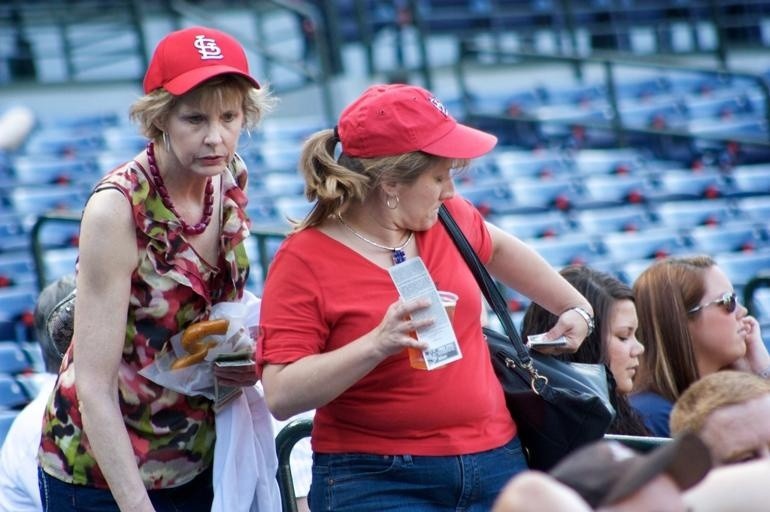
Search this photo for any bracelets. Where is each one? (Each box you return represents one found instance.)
[560,305,596,337]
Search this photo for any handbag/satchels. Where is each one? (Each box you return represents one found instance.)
[481,326,615,472]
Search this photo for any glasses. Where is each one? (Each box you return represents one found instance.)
[690,294,738,312]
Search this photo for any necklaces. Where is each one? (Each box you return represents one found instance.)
[337,208,413,266]
[146,141,215,236]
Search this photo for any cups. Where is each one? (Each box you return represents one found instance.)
[399,290,458,371]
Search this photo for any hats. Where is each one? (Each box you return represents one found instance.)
[143,27,261,97]
[33,275,76,361]
[553,429,714,510]
[336,84,498,160]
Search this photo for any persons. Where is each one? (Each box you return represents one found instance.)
[0,272,77,511]
[629,256,770,403]
[492,426,770,512]
[37,27,281,511]
[255,83,596,512]
[668,370,770,468]
[521,262,656,436]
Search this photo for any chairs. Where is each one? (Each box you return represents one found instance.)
[235,98,348,300]
